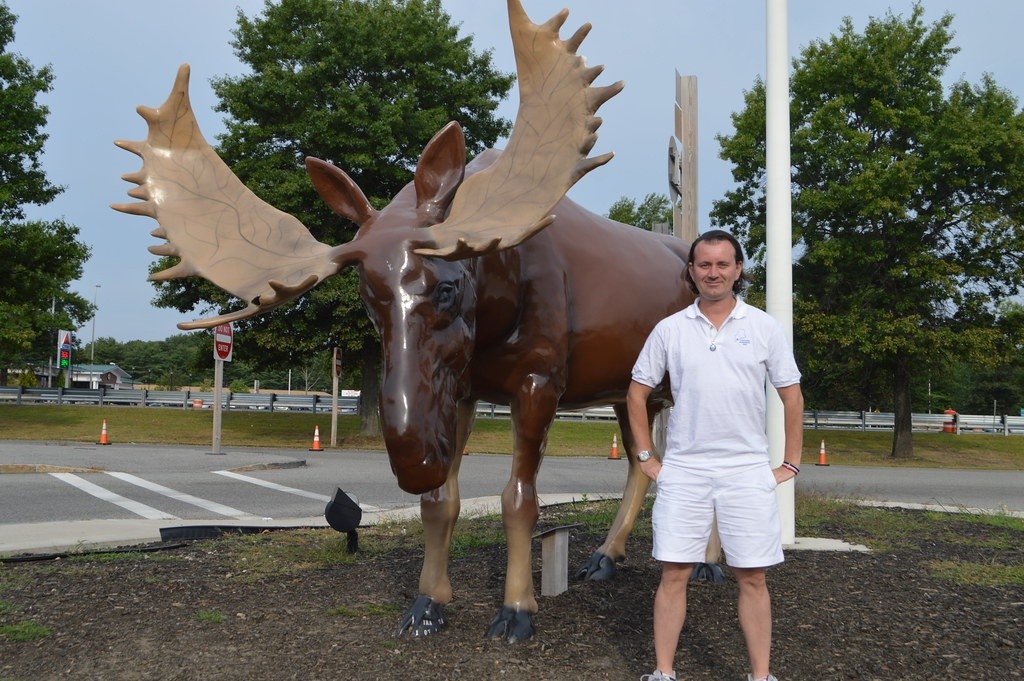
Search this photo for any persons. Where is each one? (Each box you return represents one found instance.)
[626,230,804,681]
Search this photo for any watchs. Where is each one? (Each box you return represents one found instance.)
[636,450,654,461]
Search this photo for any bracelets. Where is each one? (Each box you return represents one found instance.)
[781,461,799,475]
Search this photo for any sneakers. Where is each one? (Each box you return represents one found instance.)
[747,672,778,681]
[640,670,677,681]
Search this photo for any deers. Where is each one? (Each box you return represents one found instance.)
[108,1,742,646]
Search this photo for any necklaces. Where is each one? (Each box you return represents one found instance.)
[697,317,735,351]
